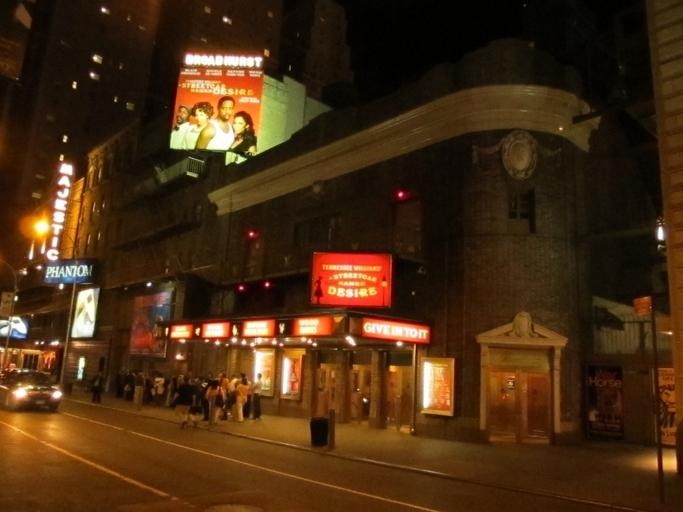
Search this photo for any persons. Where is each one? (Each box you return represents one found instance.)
[89,370,104,402]
[195,96,234,151]
[170,104,187,150]
[112,364,262,429]
[182,101,214,150]
[228,111,256,154]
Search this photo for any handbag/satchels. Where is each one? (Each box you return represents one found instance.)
[214,394,223,408]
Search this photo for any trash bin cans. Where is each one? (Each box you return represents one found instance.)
[310,417,328,446]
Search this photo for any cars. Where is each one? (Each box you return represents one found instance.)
[0,367,63,413]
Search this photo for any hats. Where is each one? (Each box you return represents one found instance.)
[178,105,191,113]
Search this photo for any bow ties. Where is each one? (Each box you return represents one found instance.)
[174,125,180,130]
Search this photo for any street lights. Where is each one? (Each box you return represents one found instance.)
[29,219,81,399]
[652,220,667,511]
